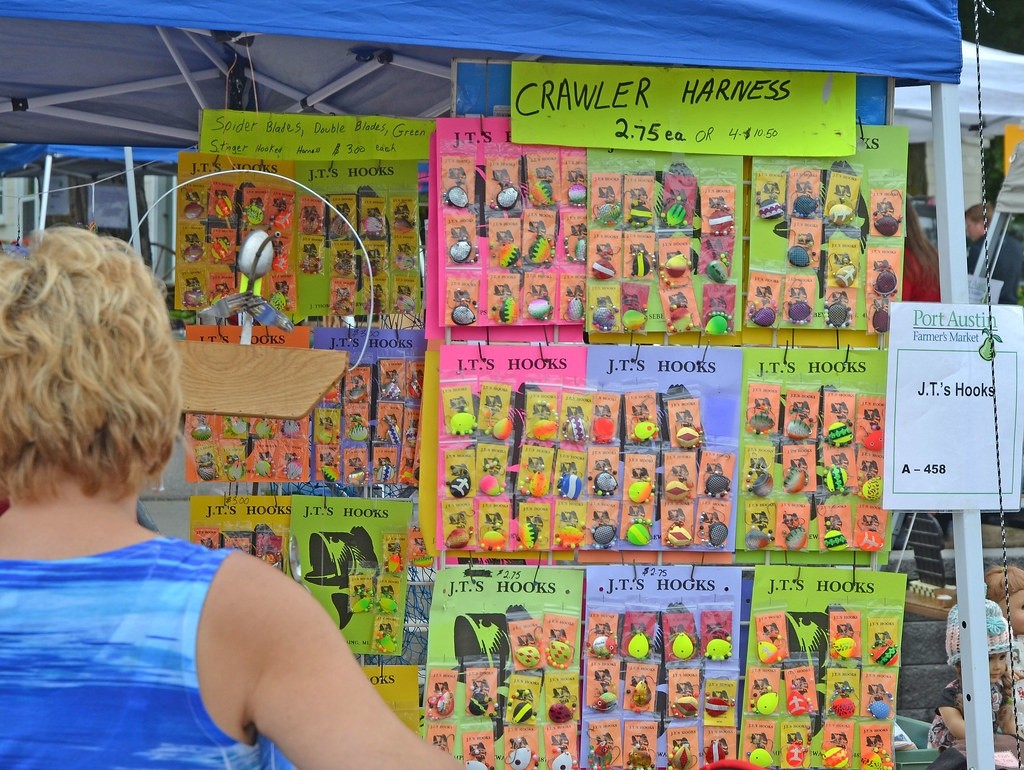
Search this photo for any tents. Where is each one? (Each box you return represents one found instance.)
[894,39,1024,143]
[0,0,995,770]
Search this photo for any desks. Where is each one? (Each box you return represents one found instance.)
[905,587,957,619]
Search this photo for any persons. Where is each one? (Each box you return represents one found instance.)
[927,583,1024,750]
[902,196,940,302]
[0,226,467,770]
[984,566,1024,735]
[965,202,1022,304]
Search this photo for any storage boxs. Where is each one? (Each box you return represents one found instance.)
[896,715,944,770]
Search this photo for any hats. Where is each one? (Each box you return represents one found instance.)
[944,583,1014,666]
